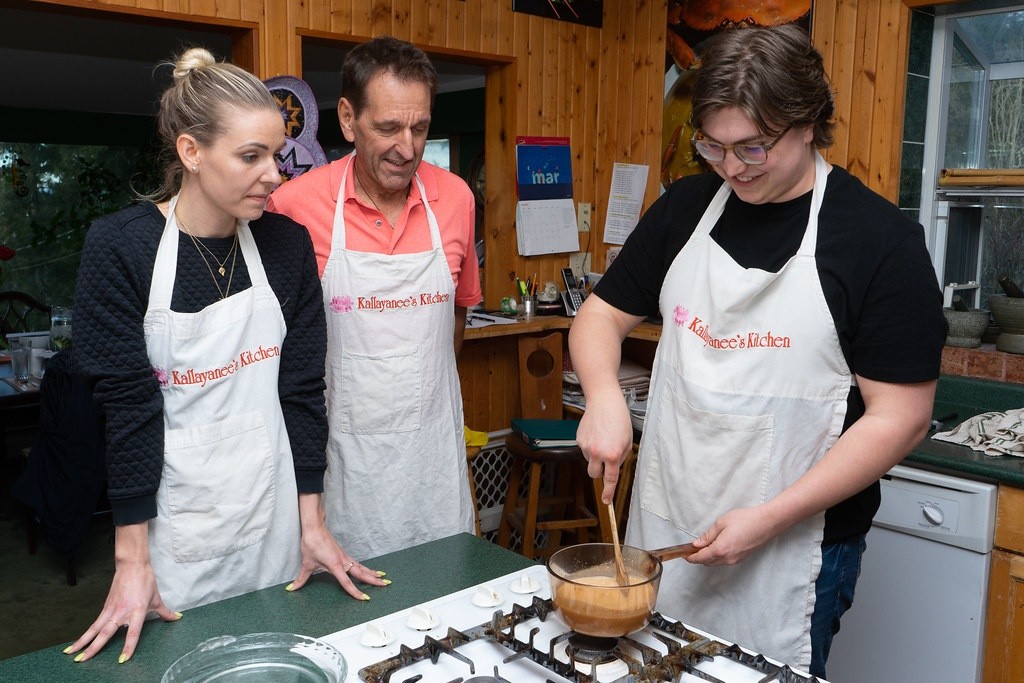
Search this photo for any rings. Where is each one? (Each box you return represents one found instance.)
[109,620,119,627]
[349,562,354,572]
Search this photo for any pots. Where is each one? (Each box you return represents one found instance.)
[544,543,704,637]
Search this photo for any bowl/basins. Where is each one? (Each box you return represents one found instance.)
[159,630,347,683]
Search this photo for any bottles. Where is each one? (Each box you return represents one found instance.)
[48,305,74,351]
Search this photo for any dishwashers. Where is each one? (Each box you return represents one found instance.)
[823,465,997,683]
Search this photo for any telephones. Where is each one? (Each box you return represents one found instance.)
[559,268,589,317]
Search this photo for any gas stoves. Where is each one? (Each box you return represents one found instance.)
[288,562,832,683]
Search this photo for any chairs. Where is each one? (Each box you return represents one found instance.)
[7,345,119,587]
[0,290,54,352]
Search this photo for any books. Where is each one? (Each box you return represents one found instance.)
[562,358,650,405]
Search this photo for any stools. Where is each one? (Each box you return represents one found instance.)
[465,444,483,537]
[495,432,615,563]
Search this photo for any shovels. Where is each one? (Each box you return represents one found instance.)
[601,472,629,597]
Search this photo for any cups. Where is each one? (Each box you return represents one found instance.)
[522,301,536,317]
[9,338,33,383]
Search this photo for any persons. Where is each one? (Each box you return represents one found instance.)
[568,26,950,678]
[61,41,392,662]
[264,37,484,562]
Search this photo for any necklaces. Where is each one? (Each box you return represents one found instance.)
[176,209,238,300]
[353,160,381,213]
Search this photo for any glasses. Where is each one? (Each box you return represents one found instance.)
[464,314,495,326]
[689,124,794,166]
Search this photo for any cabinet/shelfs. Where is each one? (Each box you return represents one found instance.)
[978,485,1024,683]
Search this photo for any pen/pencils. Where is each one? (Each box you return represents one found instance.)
[533,283,538,296]
[526,276,531,289]
[530,273,537,296]
[516,278,523,295]
[470,315,496,322]
[575,274,595,291]
